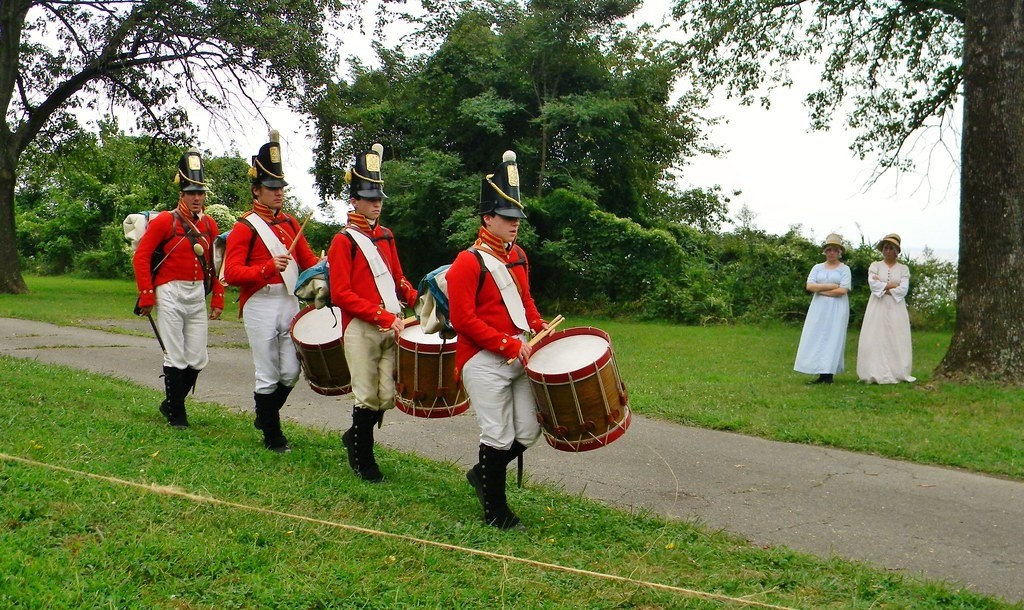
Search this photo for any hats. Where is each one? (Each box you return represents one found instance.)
[477,150,527,220]
[247,129,289,189]
[822,233,845,253]
[877,233,901,254]
[345,142,389,200]
[174,147,210,192]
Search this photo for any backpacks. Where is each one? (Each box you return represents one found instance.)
[293,225,390,310]
[119,210,210,261]
[212,214,295,288]
[414,245,526,341]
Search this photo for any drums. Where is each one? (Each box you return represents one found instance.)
[289,303,352,396]
[392,315,472,418]
[521,326,632,452]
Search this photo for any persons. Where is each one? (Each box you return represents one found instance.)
[446,150,555,529]
[793,232,851,385]
[224,131,319,453]
[856,233,916,385]
[132,147,224,428]
[327,144,420,484]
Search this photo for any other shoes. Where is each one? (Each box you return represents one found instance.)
[807,373,834,386]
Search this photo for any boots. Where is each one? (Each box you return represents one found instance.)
[158,366,202,419]
[465,440,527,508]
[342,409,385,473]
[162,365,191,428]
[479,444,520,531]
[352,404,386,484]
[254,392,290,452]
[254,381,294,430]
